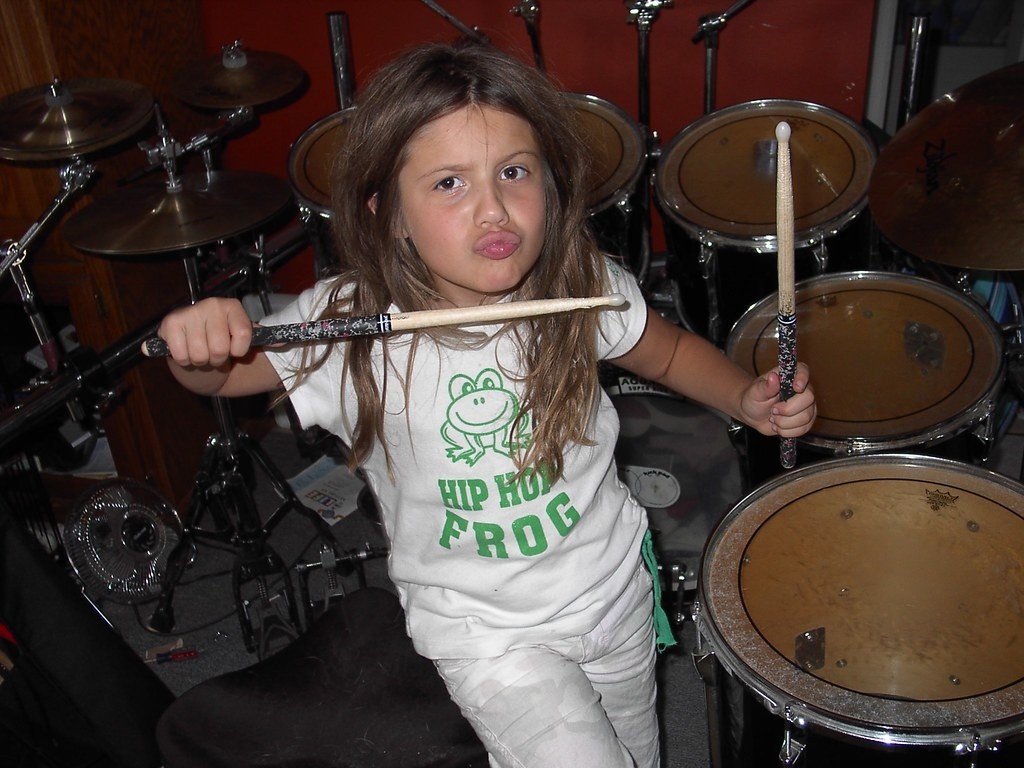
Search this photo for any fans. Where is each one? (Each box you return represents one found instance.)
[64,479,189,606]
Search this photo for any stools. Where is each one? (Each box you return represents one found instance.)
[155,588,491,768]
[610,392,741,768]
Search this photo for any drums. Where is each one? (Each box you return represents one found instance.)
[722,270,1009,496]
[689,453,1024,768]
[648,97,881,353]
[286,103,368,277]
[553,89,664,286]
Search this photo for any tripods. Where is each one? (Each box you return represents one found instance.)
[146,247,354,635]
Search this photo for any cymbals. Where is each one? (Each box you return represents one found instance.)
[866,58,1024,271]
[61,166,293,256]
[0,79,158,162]
[170,47,307,110]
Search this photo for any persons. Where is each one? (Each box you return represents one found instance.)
[157,43,819,768]
